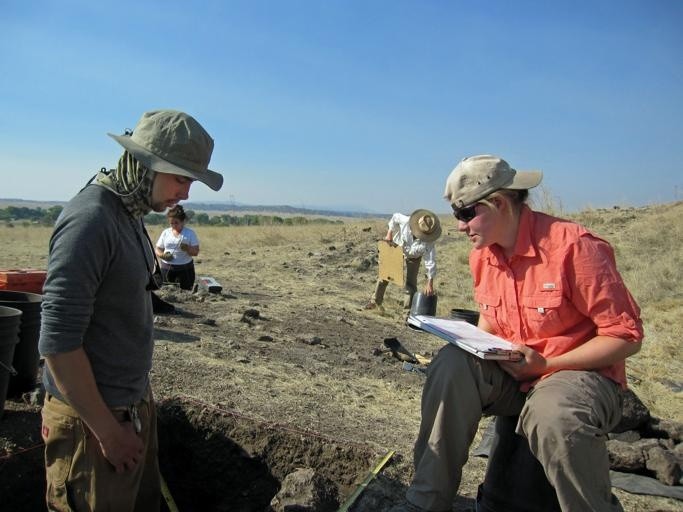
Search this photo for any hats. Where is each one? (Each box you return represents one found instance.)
[408,209,442,242]
[444,155,543,210]
[107,109,224,192]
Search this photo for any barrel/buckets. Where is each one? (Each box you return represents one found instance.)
[412,290,437,319]
[475,415,559,511]
[0,305,22,430]
[1,289,45,397]
[450,309,479,327]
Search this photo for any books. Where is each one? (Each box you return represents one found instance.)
[406,313,524,361]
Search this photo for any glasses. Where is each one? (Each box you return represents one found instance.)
[454,197,489,222]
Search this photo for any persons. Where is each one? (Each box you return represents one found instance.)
[153,204,200,291]
[391,154,644,512]
[363,209,442,309]
[36,109,223,512]
